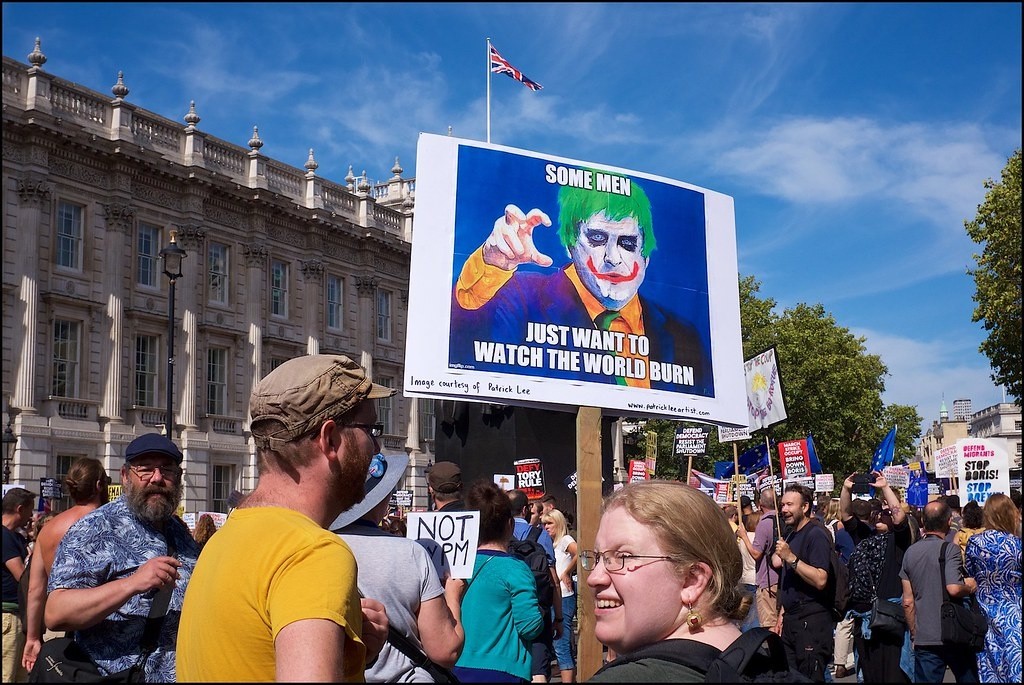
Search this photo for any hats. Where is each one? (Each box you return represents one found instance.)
[428,461,462,493]
[327,451,409,531]
[125,432,183,465]
[249,354,400,452]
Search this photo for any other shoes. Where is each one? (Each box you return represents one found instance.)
[833,664,855,678]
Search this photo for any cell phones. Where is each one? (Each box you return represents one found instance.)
[853,474,876,485]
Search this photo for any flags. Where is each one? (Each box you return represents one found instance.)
[714,438,770,480]
[868,427,895,496]
[806,433,823,473]
[488,42,544,92]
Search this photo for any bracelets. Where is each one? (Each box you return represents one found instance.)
[791,557,799,569]
[555,618,564,623]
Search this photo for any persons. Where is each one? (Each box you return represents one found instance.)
[378,505,407,538]
[2,456,111,684]
[583,480,814,684]
[329,452,466,684]
[43,432,200,683]
[428,461,577,683]
[192,514,217,551]
[723,470,1022,684]
[175,355,401,684]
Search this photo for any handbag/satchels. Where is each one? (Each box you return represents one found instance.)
[940,600,988,654]
[27,637,146,685]
[867,595,907,641]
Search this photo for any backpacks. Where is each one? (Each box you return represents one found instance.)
[785,520,855,622]
[761,513,792,598]
[505,526,554,608]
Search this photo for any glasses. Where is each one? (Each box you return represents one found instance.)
[93,477,112,485]
[518,503,534,514]
[310,421,386,440]
[579,550,689,571]
[127,466,183,481]
[873,508,892,515]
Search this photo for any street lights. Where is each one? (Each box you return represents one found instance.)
[2,420,18,484]
[159,229,189,441]
